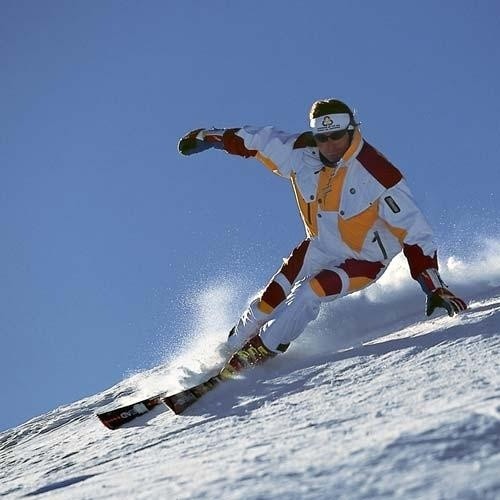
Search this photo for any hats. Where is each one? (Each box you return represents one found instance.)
[310,99,355,136]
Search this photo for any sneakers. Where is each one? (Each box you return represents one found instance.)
[219,335,278,381]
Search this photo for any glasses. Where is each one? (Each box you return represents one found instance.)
[315,129,347,143]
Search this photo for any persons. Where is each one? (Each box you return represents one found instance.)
[179,100,469,394]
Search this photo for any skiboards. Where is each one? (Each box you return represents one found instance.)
[97,373,227,430]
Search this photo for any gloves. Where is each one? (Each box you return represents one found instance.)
[417,267,467,317]
[178,128,213,155]
